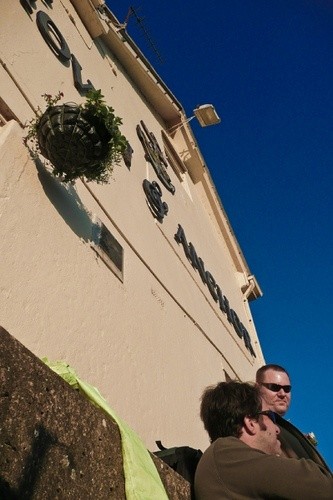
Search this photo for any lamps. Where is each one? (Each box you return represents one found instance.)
[167,103,220,135]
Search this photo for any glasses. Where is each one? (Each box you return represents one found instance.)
[260,382,292,393]
[256,409,276,424]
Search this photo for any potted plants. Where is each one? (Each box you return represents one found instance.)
[21,86,132,185]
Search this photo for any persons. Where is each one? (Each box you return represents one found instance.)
[192,382,333,500]
[253,364,332,480]
